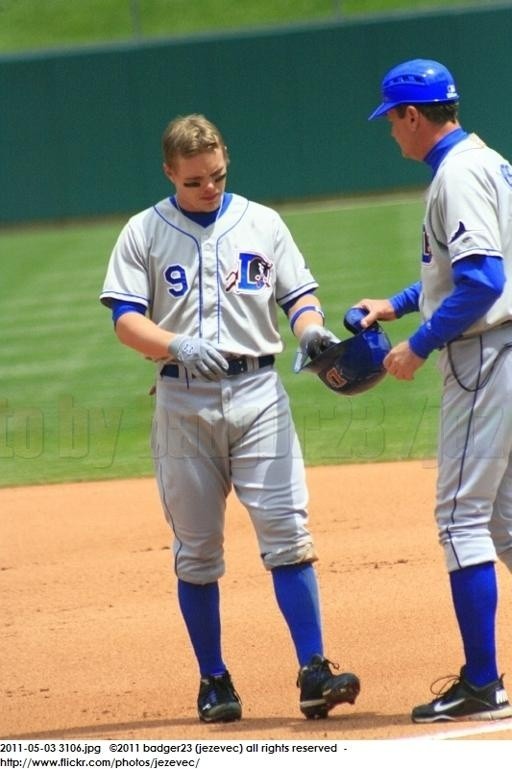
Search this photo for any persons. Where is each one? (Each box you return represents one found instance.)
[351,55,511,723]
[101,113,362,723]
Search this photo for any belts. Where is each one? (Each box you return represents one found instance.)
[157,355,274,382]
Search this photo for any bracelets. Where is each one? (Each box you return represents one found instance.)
[289,304,326,336]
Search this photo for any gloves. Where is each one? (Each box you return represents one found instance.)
[291,321,342,375]
[166,332,229,385]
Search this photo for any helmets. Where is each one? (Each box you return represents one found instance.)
[294,308,392,397]
[367,55,459,122]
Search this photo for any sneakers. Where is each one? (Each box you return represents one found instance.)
[195,671,243,722]
[410,663,512,722]
[293,653,361,720]
